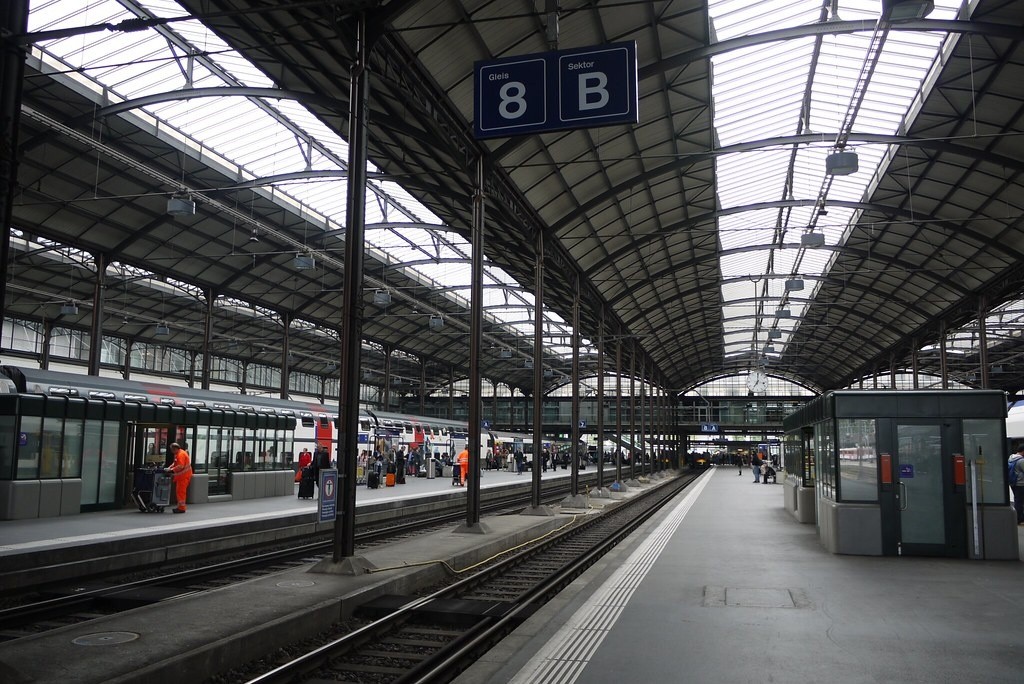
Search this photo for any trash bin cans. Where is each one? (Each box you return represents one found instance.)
[131,461,174,513]
[452,458,461,486]
[426,457,436,479]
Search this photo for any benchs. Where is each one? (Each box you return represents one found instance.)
[442,466,453,477]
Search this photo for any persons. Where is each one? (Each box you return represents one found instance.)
[440,452,450,467]
[751,450,776,484]
[405,446,425,477]
[486,447,509,471]
[373,445,384,488]
[457,447,468,486]
[604,451,657,465]
[387,445,397,474]
[396,446,407,484]
[307,443,331,489]
[298,448,312,469]
[542,448,570,472]
[712,452,750,466]
[147,443,154,455]
[164,443,193,513]
[514,447,523,475]
[737,453,743,475]
[1008,445,1024,525]
[360,450,372,463]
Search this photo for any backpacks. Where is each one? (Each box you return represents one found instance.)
[1008,457,1024,485]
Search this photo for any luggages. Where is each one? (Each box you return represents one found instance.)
[298,466,314,499]
[386,463,395,486]
[367,462,378,489]
[561,461,566,468]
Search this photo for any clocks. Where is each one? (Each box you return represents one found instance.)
[746,370,769,393]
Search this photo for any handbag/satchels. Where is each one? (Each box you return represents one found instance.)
[758,459,763,466]
[295,470,302,482]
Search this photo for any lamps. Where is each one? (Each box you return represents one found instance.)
[441,389,448,394]
[373,290,391,305]
[154,323,170,336]
[757,228,826,366]
[294,250,315,270]
[360,369,368,379]
[586,386,592,393]
[560,376,569,383]
[544,369,553,376]
[429,315,444,328]
[880,0,935,24]
[826,149,859,175]
[524,359,534,367]
[288,353,293,361]
[227,342,239,350]
[327,363,337,371]
[501,348,511,358]
[393,377,401,385]
[166,192,195,216]
[61,302,79,316]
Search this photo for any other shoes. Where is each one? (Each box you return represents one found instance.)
[761,482,768,484]
[460,484,464,487]
[1017,520,1024,526]
[753,481,759,483]
[172,508,185,513]
